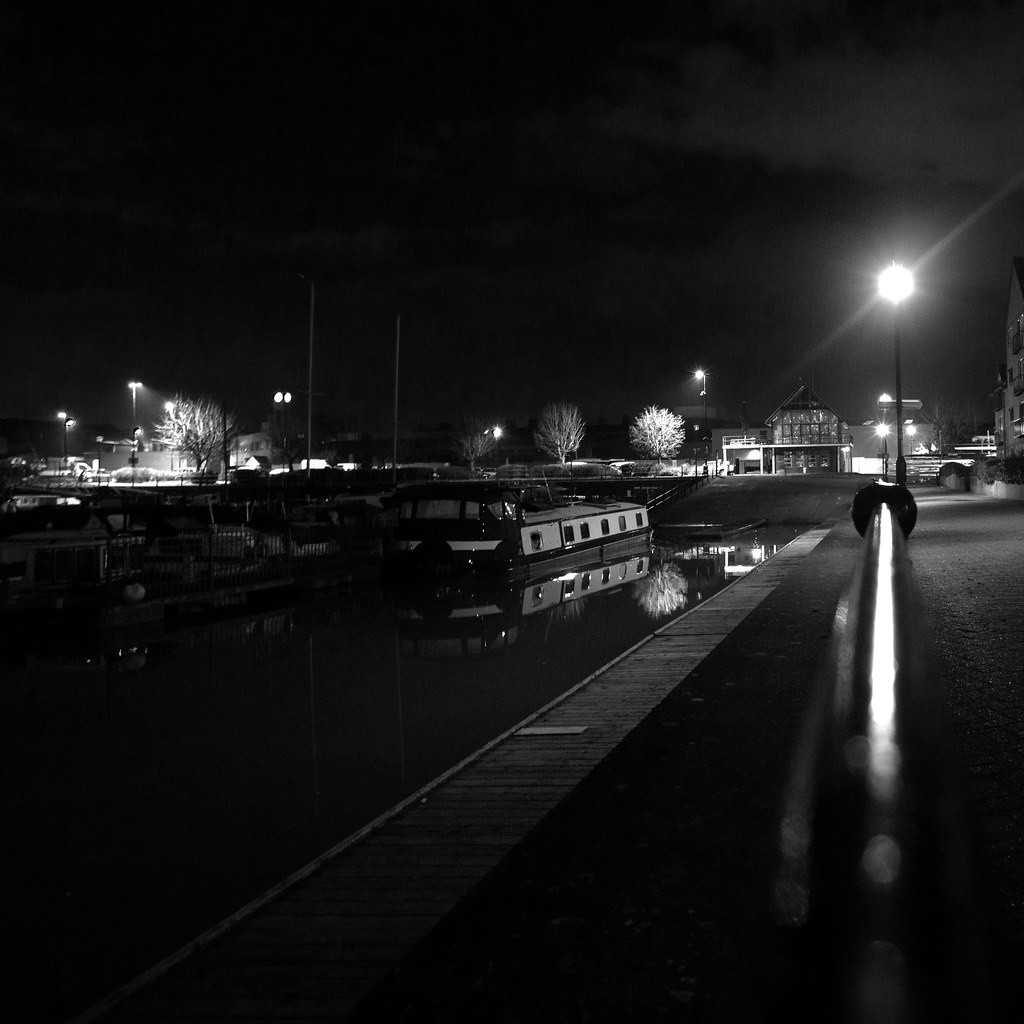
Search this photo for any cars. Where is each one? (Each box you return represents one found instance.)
[480,467,498,480]
[81,467,112,483]
[698,460,722,476]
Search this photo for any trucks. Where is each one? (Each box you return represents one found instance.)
[38,462,91,479]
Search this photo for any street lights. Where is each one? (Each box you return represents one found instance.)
[491,428,503,479]
[694,371,709,475]
[273,391,293,464]
[876,423,889,482]
[64,418,76,456]
[128,382,143,484]
[906,426,916,458]
[96,435,104,484]
[867,244,922,487]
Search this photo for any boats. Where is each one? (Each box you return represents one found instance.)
[0,473,655,608]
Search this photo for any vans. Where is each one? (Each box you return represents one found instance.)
[301,459,332,470]
[607,462,635,469]
